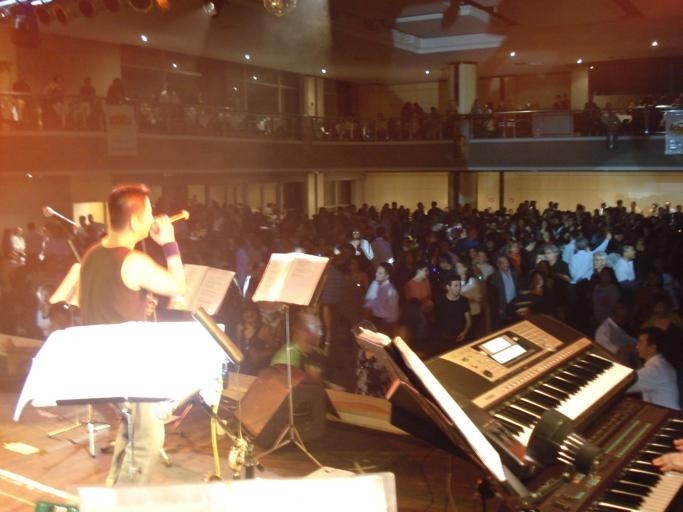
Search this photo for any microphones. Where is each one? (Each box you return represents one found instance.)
[42,205,81,231]
[151,208,189,231]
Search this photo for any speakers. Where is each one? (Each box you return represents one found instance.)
[233,362,328,444]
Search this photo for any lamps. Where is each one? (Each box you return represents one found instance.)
[263,0,298,17]
[0,0,228,34]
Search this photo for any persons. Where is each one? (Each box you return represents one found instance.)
[623,327,679,412]
[653,437,682,475]
[2,186,682,386]
[325,93,681,136]
[73,184,192,488]
[1,65,302,137]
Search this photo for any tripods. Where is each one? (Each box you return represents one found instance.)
[47,404,116,458]
[253,310,324,472]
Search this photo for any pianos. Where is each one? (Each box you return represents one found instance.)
[420,317,683,512]
